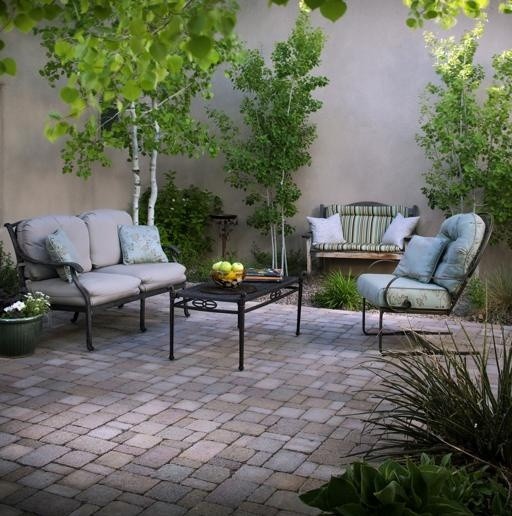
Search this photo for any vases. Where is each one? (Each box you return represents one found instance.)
[0,313,44,357]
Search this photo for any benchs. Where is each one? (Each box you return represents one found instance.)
[2,207,191,353]
[301,200,422,280]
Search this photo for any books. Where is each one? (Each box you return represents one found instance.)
[242,268,284,283]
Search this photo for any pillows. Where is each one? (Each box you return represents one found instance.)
[379,211,422,251]
[305,212,348,246]
[116,223,170,267]
[391,234,450,285]
[46,223,83,283]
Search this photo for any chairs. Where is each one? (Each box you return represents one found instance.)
[356,211,495,357]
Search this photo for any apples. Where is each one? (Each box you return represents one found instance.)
[211,261,244,280]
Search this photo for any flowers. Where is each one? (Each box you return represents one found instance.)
[0,241,50,317]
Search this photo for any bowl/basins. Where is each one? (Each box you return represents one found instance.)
[210,270,247,289]
